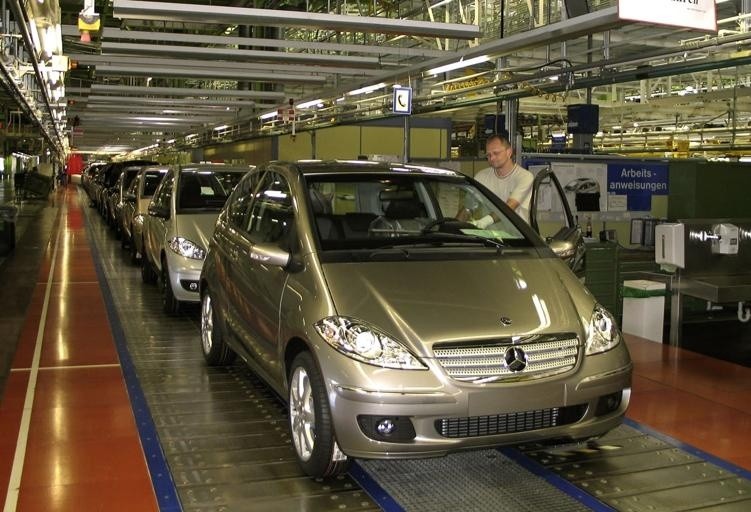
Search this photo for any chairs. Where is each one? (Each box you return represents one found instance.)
[368,189,440,239]
[285,190,341,252]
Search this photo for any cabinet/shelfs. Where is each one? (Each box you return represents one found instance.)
[574,245,706,324]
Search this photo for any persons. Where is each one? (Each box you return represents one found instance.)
[455,133,533,239]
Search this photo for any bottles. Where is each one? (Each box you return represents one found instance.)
[586,216,593,238]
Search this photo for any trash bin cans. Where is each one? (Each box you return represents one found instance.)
[0,206,17,256]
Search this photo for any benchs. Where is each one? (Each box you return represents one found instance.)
[265,212,386,240]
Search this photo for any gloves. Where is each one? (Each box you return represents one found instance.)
[466,214,495,231]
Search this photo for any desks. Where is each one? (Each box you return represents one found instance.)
[634,268,751,347]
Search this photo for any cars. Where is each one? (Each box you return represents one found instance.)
[117,165,172,263]
[81,160,160,240]
[200,156,634,482]
[147,164,333,314]
